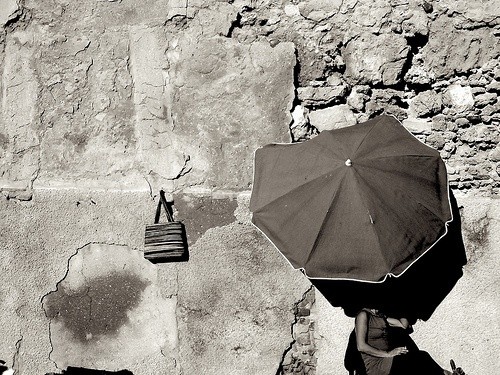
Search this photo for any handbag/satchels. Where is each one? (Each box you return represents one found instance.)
[140,189,189,262]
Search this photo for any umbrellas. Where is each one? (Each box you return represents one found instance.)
[246,111,453,284]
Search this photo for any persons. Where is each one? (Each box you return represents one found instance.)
[355,285,466,375]
